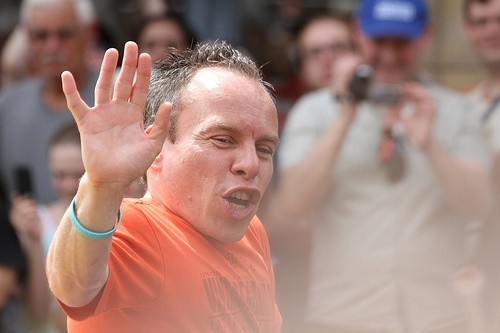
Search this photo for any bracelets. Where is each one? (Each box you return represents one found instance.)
[69,199,121,238]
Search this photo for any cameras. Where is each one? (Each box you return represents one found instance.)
[336,60,404,104]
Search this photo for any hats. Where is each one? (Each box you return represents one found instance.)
[359,0,429,38]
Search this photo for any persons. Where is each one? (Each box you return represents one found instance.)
[0,0,500,333]
